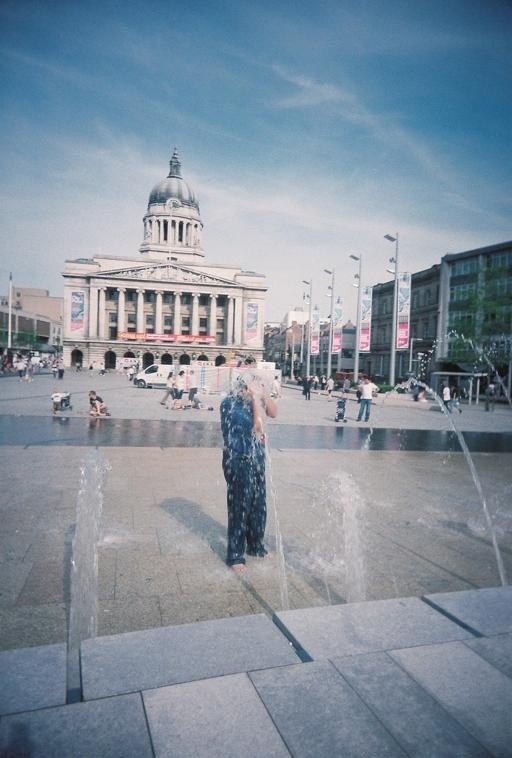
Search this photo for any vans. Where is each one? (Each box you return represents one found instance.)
[132,363,190,390]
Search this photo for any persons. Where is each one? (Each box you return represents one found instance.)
[137,360,141,372]
[160,372,176,406]
[439,381,464,413]
[88,390,112,416]
[295,373,334,402]
[412,385,425,402]
[88,361,97,377]
[75,359,82,376]
[51,390,72,415]
[218,357,281,577]
[334,375,380,423]
[122,359,139,381]
[185,370,198,409]
[1,353,65,384]
[166,369,185,410]
[99,362,105,376]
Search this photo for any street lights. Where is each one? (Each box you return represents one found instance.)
[283,328,293,374]
[382,229,401,388]
[348,251,363,380]
[322,265,337,380]
[301,278,313,377]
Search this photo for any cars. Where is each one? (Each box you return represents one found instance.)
[335,370,369,380]
